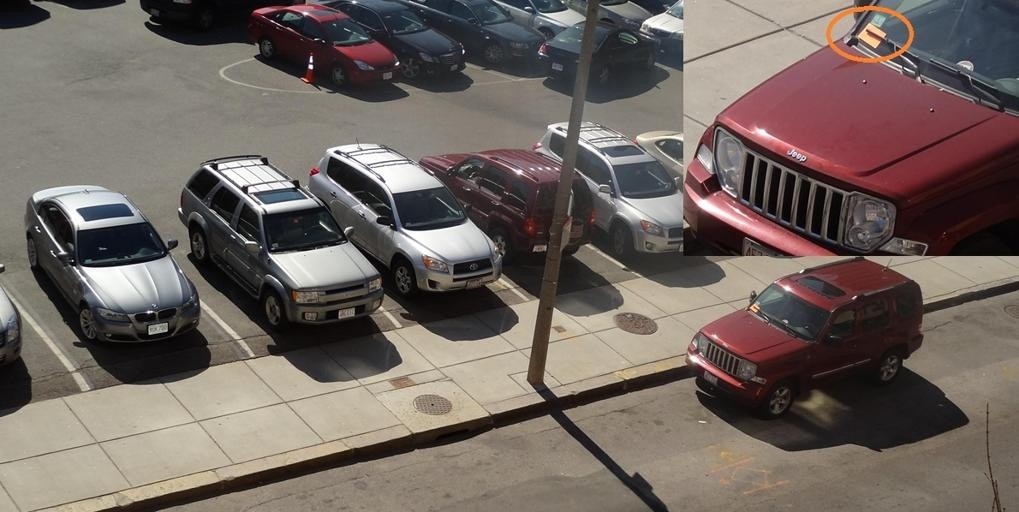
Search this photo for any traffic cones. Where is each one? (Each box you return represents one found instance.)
[301,52,319,83]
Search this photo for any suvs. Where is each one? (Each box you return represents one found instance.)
[684,2,1017,258]
[688,257,925,421]
[177,153,385,330]
[531,117,685,266]
[310,136,504,299]
[418,147,594,264]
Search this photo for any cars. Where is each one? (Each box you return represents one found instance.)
[635,128,683,184]
[138,0,240,39]
[538,20,662,87]
[562,0,653,31]
[246,2,402,88]
[639,1,683,59]
[22,183,201,347]
[494,0,587,39]
[406,0,547,70]
[0,262,23,368]
[323,0,468,83]
